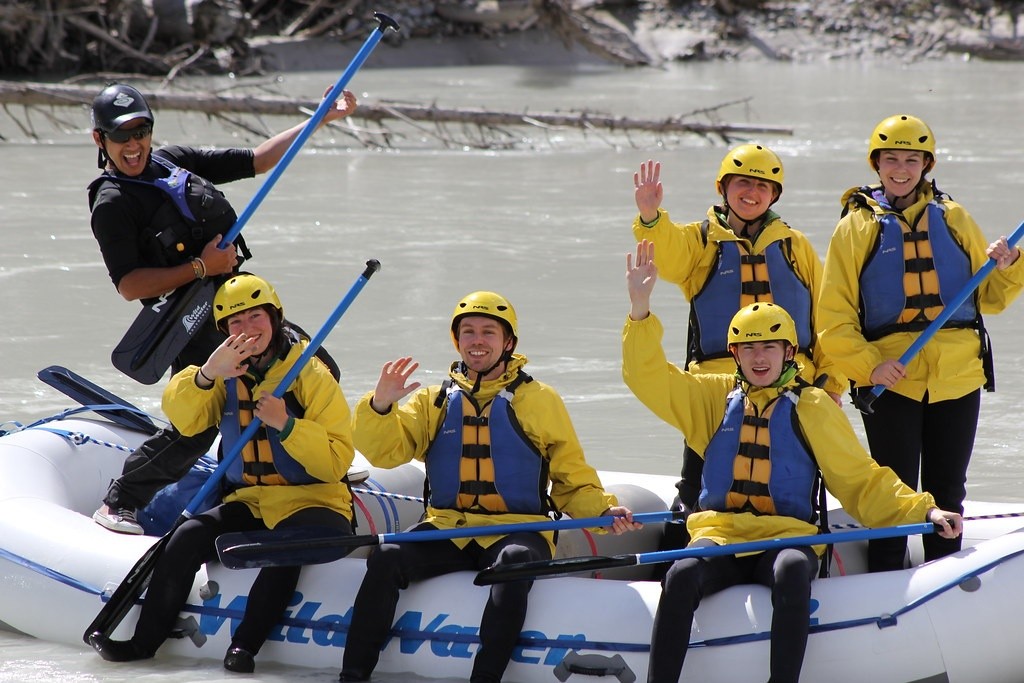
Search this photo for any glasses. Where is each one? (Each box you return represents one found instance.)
[100,123,151,143]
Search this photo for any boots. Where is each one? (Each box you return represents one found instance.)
[89,603,168,662]
[224,612,274,672]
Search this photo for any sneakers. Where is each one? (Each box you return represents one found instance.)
[93,503,144,535]
[346,464,369,483]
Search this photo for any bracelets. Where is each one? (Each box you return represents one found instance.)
[190,260,201,280]
[199,368,215,381]
[194,257,206,280]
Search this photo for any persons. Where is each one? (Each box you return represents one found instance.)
[89,276,357,673]
[817,113,1023,573]
[618,238,963,683]
[633,141,848,584]
[86,80,357,534]
[339,290,644,682]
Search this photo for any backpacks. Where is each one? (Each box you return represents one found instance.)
[86,152,253,261]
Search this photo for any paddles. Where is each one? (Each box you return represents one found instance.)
[110,11,402,387]
[215,511,686,570]
[36,365,218,465]
[474,519,957,586]
[82,259,381,646]
[853,220,1024,416]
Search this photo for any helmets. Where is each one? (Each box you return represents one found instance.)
[867,114,937,173]
[449,291,519,356]
[91,84,154,133]
[715,144,784,197]
[213,274,284,334]
[726,302,799,357]
